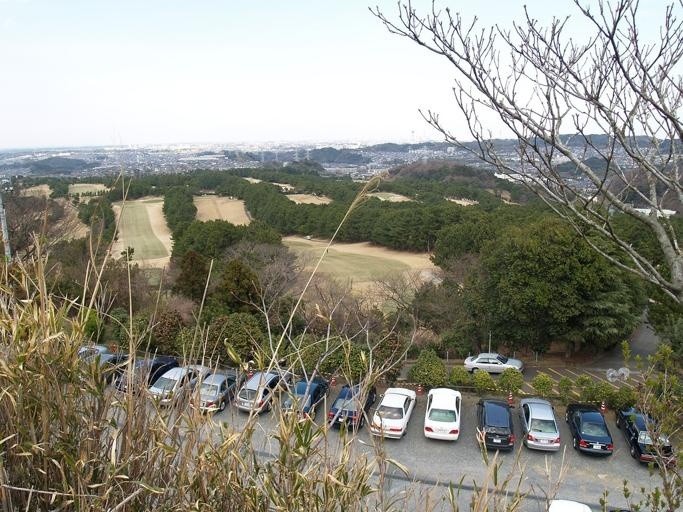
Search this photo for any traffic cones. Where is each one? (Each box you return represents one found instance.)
[508,390,515,408]
[601,399,606,413]
[416,382,425,395]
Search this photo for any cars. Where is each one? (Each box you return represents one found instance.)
[564,401,614,458]
[518,397,561,453]
[463,352,524,375]
[81,345,248,416]
[279,376,330,423]
[475,398,515,451]
[328,383,378,433]
[615,405,677,468]
[422,387,462,440]
[369,387,417,440]
[233,366,292,416]
